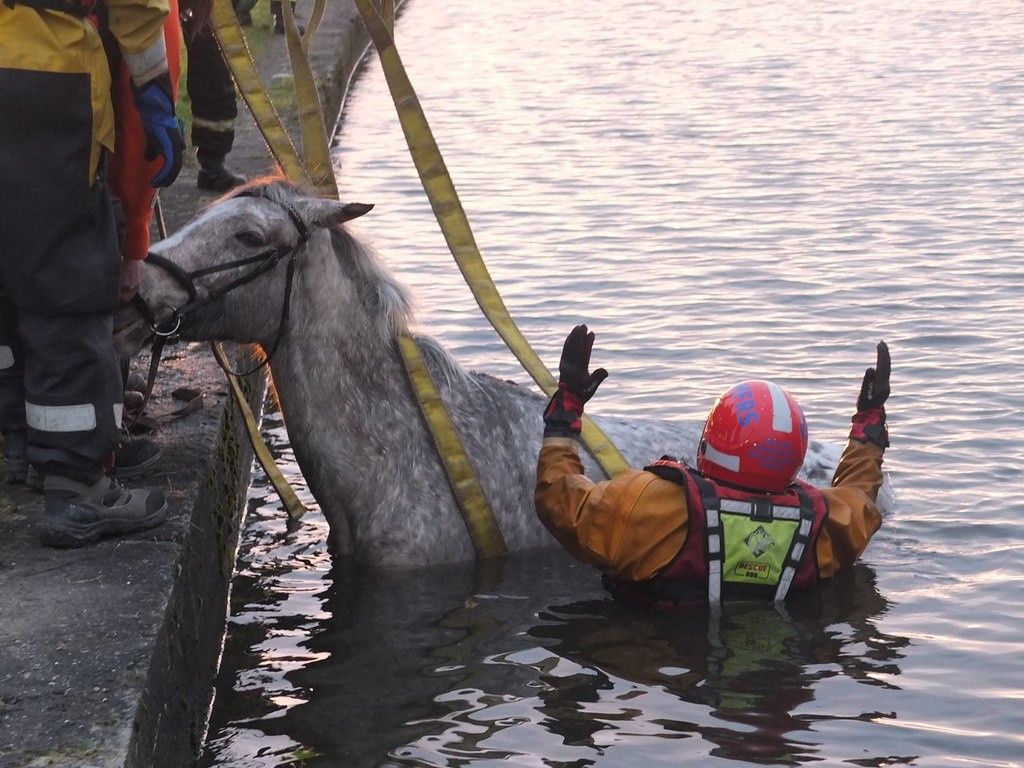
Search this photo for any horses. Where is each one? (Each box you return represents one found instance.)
[112,176,897,566]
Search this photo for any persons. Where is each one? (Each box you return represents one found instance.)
[0,0,304,547]
[533,325,891,620]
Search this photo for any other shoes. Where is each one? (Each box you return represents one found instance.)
[123,390,145,408]
[274,16,304,35]
[197,163,246,191]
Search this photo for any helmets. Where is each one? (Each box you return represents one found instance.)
[697,380,809,494]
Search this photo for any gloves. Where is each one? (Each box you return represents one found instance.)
[543,324,609,432]
[847,341,891,449]
[130,68,187,189]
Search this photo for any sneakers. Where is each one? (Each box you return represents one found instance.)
[39,475,168,547]
[1,455,25,483]
[26,438,165,492]
